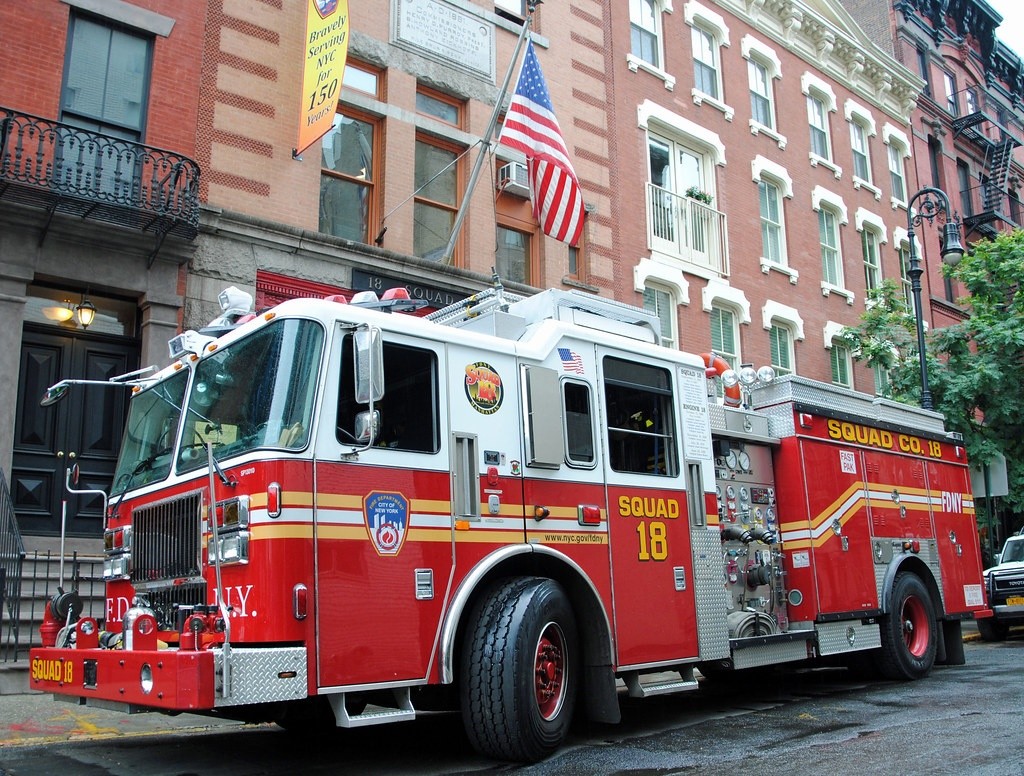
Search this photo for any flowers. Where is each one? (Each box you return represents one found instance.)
[685,185,714,204]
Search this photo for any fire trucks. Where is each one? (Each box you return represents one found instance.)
[30,265,995,766]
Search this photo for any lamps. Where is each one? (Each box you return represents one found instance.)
[76,286,97,331]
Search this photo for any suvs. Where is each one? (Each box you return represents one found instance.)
[974,534,1023,641]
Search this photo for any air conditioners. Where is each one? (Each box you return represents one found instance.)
[497,162,531,198]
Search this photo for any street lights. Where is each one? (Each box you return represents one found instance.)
[907,184,965,412]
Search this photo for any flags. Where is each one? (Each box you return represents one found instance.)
[493,38,584,250]
